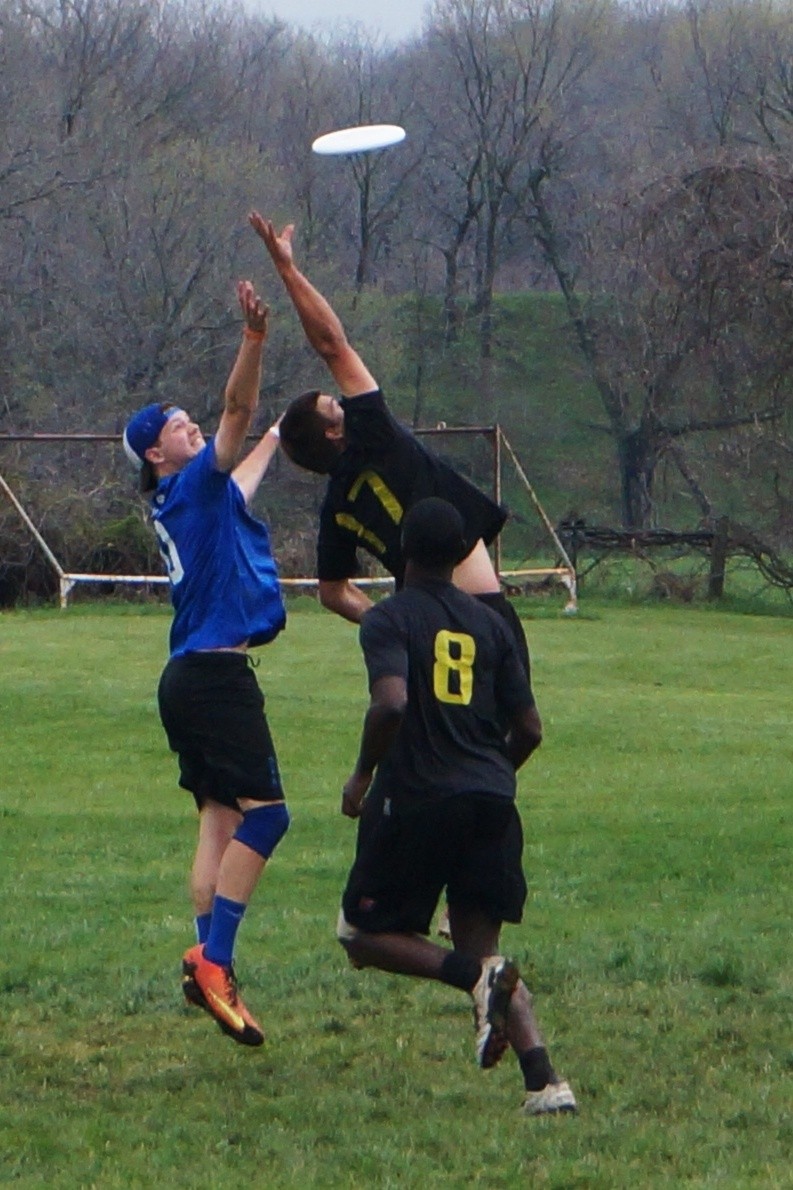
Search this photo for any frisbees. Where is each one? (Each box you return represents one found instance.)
[311,123,407,155]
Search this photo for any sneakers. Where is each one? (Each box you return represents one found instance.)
[472,951,522,1069]
[519,1079,580,1121]
[180,947,267,1048]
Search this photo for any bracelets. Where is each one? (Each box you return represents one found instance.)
[242,326,267,340]
[269,426,281,438]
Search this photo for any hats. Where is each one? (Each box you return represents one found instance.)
[401,496,466,590]
[121,398,185,493]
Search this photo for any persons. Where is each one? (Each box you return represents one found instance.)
[336,490,578,1113]
[247,209,532,943]
[124,272,288,1045]
